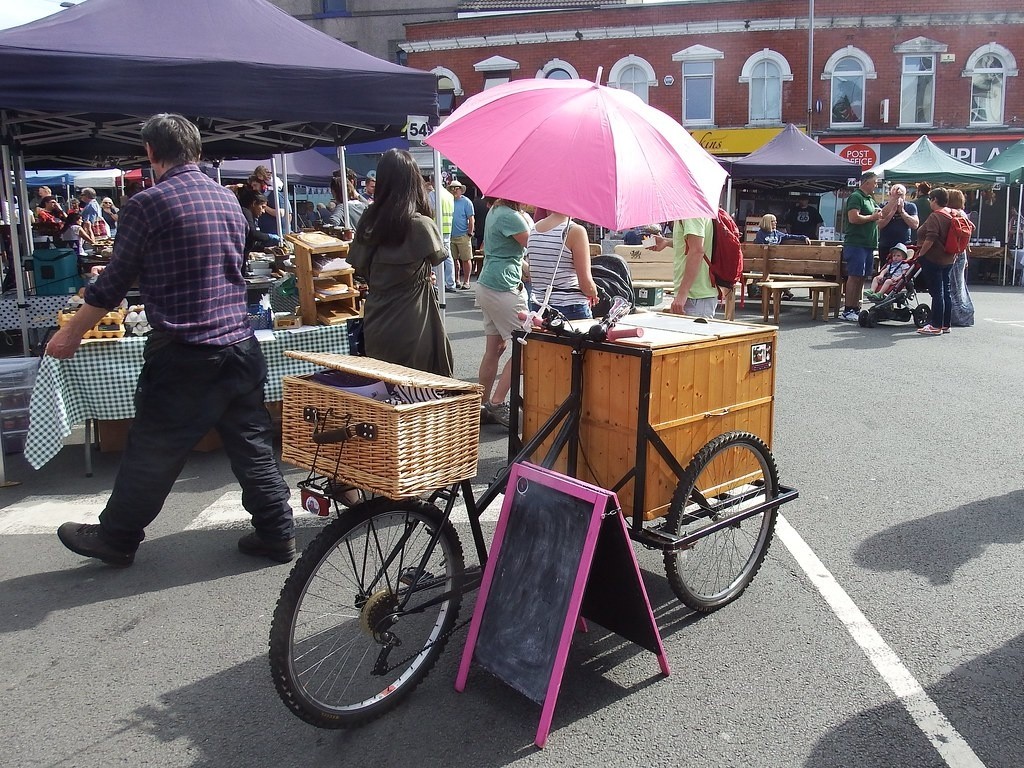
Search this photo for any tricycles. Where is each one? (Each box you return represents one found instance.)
[270,312,799,730]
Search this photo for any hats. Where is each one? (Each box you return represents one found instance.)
[915,181,931,195]
[447,181,467,194]
[890,242,908,260]
[430,172,443,182]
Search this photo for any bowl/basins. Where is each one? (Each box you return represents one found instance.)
[641,237,656,248]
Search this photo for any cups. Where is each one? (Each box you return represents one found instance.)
[875,208,881,220]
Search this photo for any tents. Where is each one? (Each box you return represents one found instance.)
[862,135,1009,286]
[0,0,460,360]
[976,138,1024,286]
[709,156,743,174]
[715,123,862,241]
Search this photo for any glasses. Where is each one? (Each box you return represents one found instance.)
[450,186,461,191]
[103,201,111,204]
[928,198,933,202]
[81,194,85,196]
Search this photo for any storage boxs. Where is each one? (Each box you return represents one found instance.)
[1,430,29,455]
[95,401,283,452]
[0,388,33,409]
[0,408,30,431]
[247,304,270,329]
[0,358,42,387]
[274,316,302,328]
[634,287,663,305]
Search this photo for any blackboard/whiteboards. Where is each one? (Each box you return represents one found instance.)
[464,462,663,707]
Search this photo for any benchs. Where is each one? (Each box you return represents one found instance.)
[589,243,601,257]
[612,245,843,311]
[781,239,844,246]
[758,281,839,324]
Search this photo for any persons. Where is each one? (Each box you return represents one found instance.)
[295,198,337,226]
[330,177,368,230]
[785,194,825,241]
[90,265,128,309]
[754,215,812,300]
[446,181,475,290]
[224,165,292,259]
[424,168,458,292]
[45,113,296,569]
[476,197,598,428]
[332,167,367,202]
[622,208,718,319]
[1009,205,1024,285]
[832,96,862,122]
[62,213,95,256]
[361,170,376,205]
[346,148,454,380]
[0,186,121,275]
[839,173,977,339]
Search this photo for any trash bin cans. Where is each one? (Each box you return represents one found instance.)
[32,248,81,295]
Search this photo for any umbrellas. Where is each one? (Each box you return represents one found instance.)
[425,66,731,231]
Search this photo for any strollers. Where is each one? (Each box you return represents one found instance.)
[858,245,930,328]
[588,254,636,319]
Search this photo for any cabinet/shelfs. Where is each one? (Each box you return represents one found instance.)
[283,230,361,325]
[744,217,762,242]
[523,311,780,520]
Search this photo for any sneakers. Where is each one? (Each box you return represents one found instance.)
[486,401,511,427]
[942,327,951,332]
[238,530,296,562]
[58,520,135,568]
[916,324,943,335]
[871,291,884,300]
[480,406,496,425]
[863,290,874,296]
[840,309,858,323]
[855,306,870,314]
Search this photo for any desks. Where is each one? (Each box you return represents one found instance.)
[965,246,1010,286]
[246,278,277,289]
[29,319,350,479]
[1010,249,1024,285]
[81,254,109,272]
[0,292,76,355]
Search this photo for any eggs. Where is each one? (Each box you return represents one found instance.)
[128,305,152,332]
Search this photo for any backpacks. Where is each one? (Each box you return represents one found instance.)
[680,207,744,311]
[935,209,974,254]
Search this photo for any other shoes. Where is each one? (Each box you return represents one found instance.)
[457,283,460,287]
[461,283,470,290]
[445,286,457,293]
